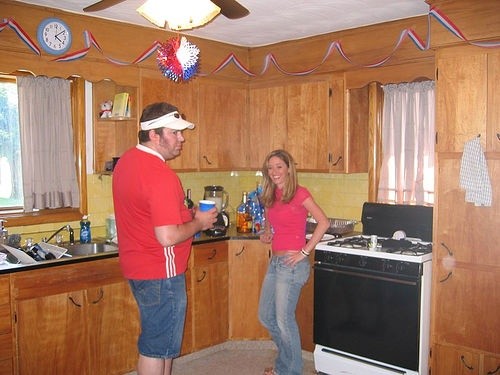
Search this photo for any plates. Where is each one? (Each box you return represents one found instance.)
[40,242,67,260]
[306,234,334,240]
[1,244,36,263]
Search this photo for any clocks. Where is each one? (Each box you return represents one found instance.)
[37,17,72,55]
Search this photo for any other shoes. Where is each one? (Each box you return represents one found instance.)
[263,367,276,375]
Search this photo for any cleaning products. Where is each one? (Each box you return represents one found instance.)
[79,214,91,244]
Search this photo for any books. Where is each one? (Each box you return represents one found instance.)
[112,93,131,118]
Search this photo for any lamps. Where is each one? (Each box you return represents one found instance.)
[136,0,221,30]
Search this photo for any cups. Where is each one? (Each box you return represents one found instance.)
[200,200,216,212]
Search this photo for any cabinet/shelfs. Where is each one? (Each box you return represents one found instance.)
[92,63,369,174]
[424,0,500,375]
[0,239,316,375]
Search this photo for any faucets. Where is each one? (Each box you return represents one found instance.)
[42,224,75,246]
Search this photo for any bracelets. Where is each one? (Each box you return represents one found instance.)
[300,248,310,258]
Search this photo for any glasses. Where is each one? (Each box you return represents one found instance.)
[150,113,187,125]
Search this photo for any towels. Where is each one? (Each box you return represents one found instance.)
[459,136,493,208]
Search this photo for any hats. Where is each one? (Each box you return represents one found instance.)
[140,110,194,130]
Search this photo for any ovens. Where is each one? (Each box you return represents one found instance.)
[312,249,432,375]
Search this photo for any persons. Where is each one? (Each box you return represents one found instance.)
[111,102,219,375]
[256,150,331,375]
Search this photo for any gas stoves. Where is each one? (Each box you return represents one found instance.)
[314,233,432,263]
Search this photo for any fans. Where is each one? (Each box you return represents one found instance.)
[82,0,250,19]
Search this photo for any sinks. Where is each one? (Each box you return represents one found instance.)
[60,242,119,257]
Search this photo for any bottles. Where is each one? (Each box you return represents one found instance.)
[186,188,193,209]
[236,191,252,233]
[79,215,91,243]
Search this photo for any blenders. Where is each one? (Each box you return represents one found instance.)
[203,186,230,238]
